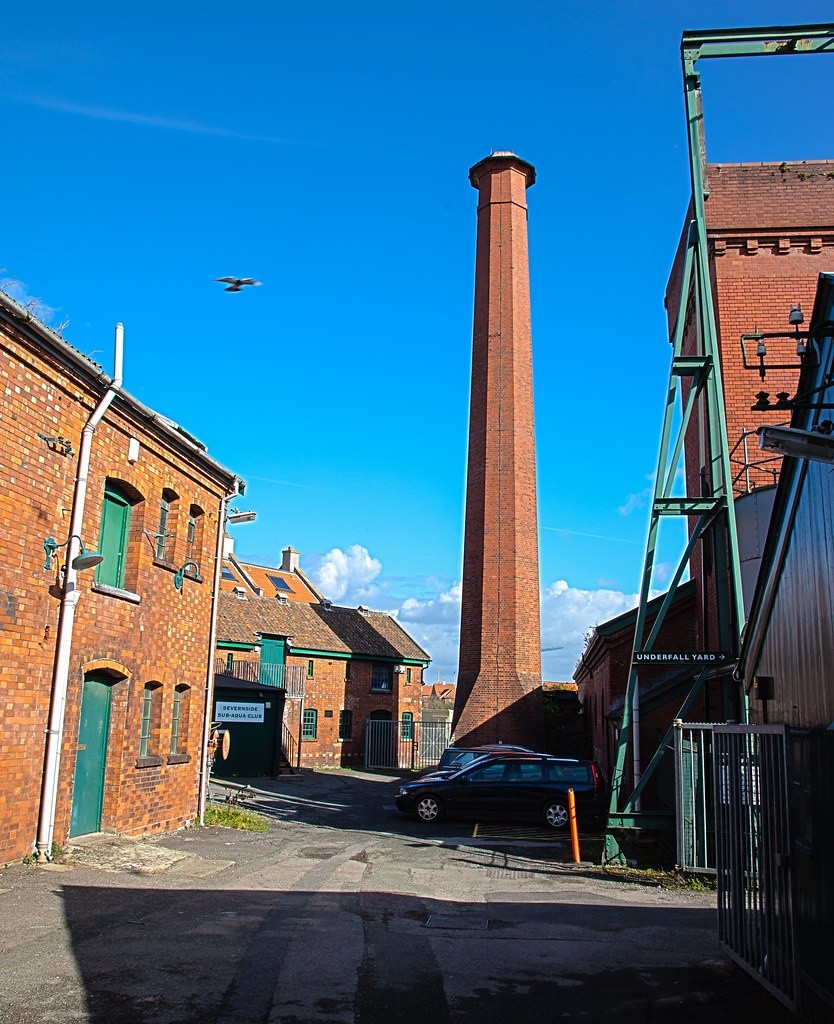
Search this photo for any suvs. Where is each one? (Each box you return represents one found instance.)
[394,743,605,830]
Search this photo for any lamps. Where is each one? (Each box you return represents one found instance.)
[43,534,104,570]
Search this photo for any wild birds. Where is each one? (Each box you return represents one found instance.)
[209,276,264,293]
